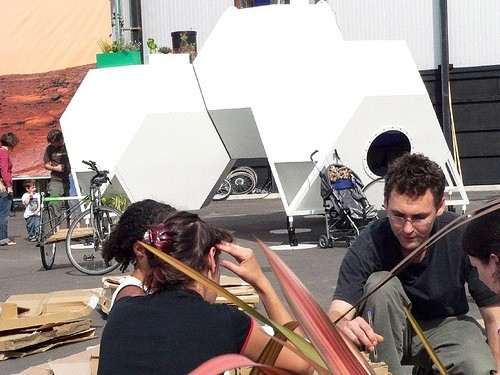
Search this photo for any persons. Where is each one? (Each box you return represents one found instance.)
[22,180,40,242]
[0,131,19,246]
[97,153,314,375]
[43,129,71,231]
[326,154,500,375]
[462,196,500,296]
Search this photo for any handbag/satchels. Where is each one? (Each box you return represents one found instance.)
[0,179,8,199]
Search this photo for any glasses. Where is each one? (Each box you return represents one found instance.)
[386,207,436,227]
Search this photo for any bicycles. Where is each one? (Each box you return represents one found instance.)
[34,160,125,275]
[211,166,258,201]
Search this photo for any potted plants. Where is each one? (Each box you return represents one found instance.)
[145,38,189,66]
[97,39,142,68]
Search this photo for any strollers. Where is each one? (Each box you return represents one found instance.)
[310,148,379,249]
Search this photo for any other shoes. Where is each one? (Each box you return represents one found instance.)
[4,241,17,245]
